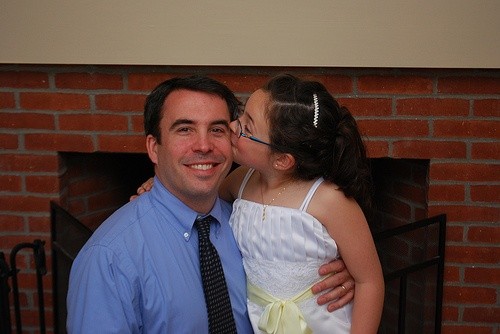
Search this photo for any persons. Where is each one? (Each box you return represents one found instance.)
[66,75,355,334]
[130,75,385,334]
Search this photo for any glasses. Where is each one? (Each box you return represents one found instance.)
[234,105,276,148]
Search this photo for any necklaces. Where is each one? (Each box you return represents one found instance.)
[259,174,294,220]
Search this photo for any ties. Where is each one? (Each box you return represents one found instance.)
[193,215,238,334]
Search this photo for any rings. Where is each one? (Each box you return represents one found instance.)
[340,285,348,294]
